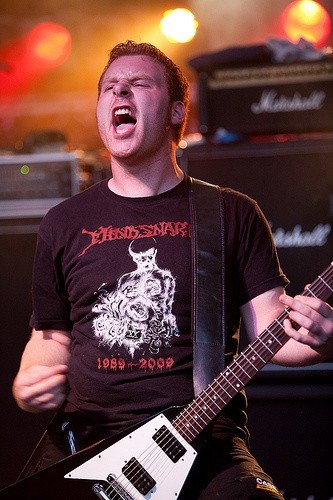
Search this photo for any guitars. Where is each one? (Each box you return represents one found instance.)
[1,260,333,500]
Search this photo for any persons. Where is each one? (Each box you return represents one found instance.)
[12,44,333,500]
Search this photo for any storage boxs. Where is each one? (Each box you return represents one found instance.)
[179,60,333,304]
[0,151,81,220]
